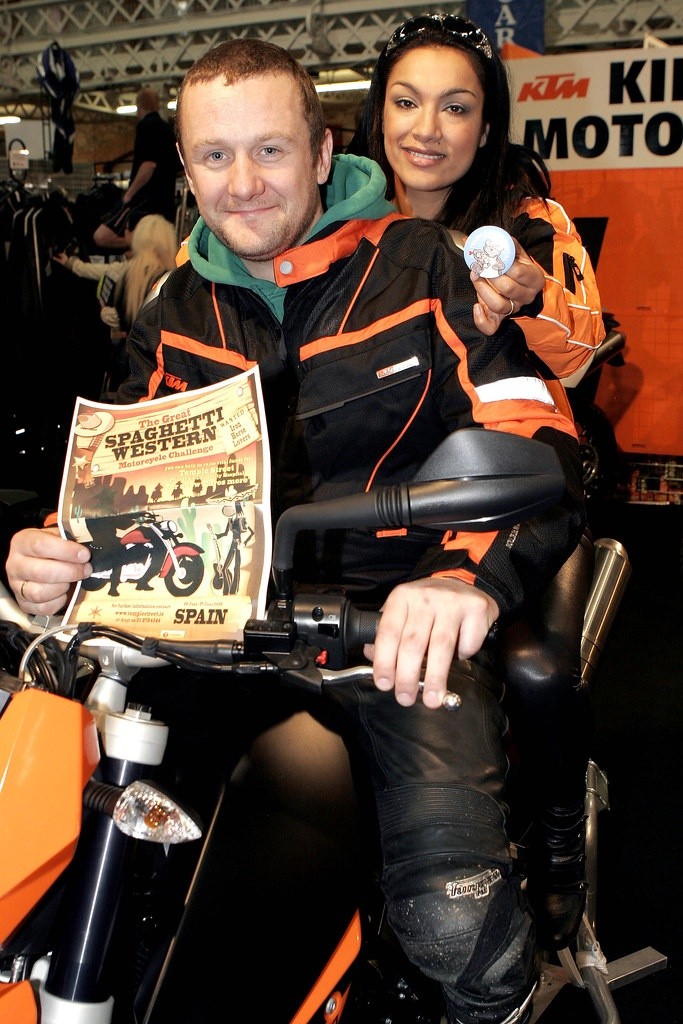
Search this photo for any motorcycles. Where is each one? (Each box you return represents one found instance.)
[3,382,670,1024]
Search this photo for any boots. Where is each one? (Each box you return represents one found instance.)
[530,795,587,949]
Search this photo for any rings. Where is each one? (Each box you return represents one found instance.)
[21,580,30,600]
[503,299,515,316]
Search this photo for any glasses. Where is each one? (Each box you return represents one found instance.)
[385,11,494,59]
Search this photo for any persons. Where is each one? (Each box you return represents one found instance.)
[344,12,605,951]
[6,39,587,1024]
[53,88,178,339]
